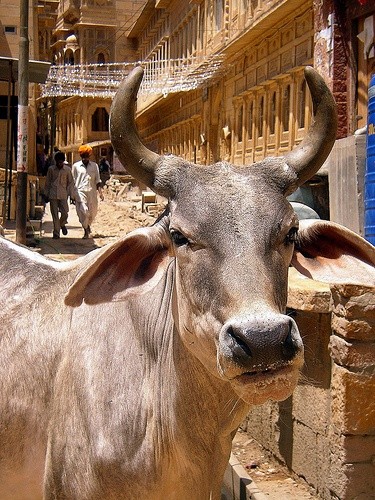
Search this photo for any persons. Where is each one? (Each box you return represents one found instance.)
[44,151,73,239]
[72,144,104,239]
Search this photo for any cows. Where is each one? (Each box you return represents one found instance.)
[1,66,375,500]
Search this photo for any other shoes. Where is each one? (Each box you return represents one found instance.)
[61,226,67,235]
[53,235,59,239]
[82,225,91,239]
